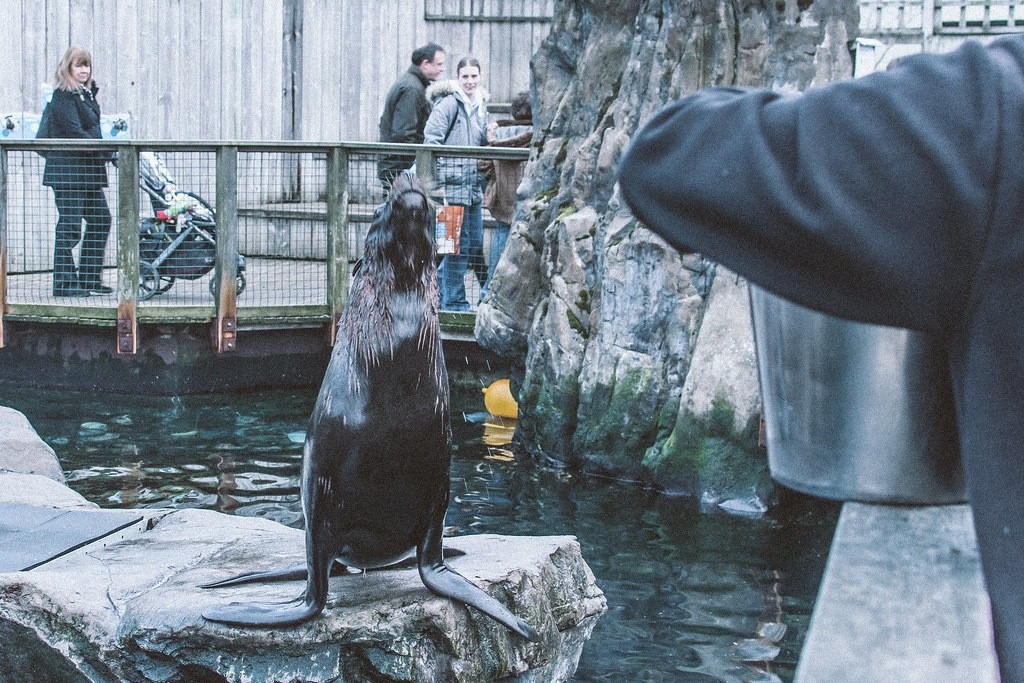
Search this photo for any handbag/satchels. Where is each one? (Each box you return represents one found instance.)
[32,102,51,157]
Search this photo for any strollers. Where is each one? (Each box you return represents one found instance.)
[111,151,247,301]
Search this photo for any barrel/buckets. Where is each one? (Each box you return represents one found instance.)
[434,205,464,255]
[752,282,974,508]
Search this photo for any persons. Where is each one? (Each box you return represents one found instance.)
[377,43,447,203]
[617,33,1024,683]
[411,57,499,313]
[43,47,113,297]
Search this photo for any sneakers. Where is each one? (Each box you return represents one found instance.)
[440,294,470,312]
[51,278,113,300]
